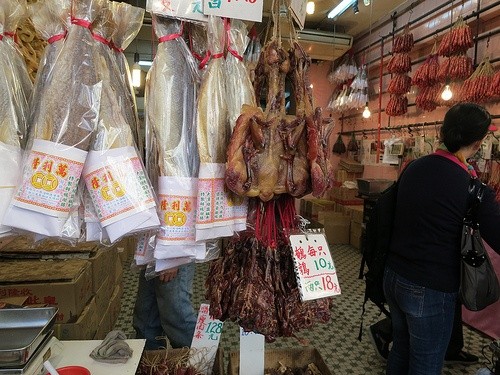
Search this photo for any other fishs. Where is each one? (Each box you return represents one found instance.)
[0,1,260,271]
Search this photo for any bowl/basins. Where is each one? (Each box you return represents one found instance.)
[46,365,90,375]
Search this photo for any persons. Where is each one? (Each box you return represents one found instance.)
[365,102,500,375]
[132,258,198,350]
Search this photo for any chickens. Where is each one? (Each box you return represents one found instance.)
[225,39,337,203]
